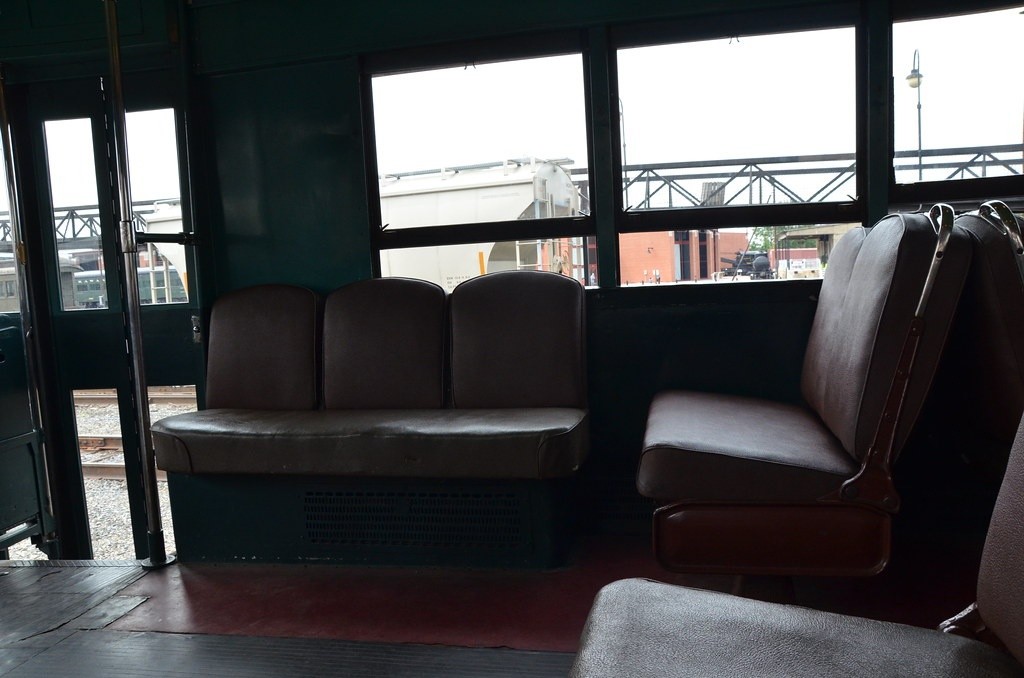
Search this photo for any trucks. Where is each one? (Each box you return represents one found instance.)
[724,250,769,276]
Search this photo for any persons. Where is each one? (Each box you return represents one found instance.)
[821,251,828,267]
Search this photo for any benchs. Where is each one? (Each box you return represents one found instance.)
[150,265,592,479]
[562,198,1024,678]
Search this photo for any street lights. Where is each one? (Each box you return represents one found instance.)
[906,49,925,181]
